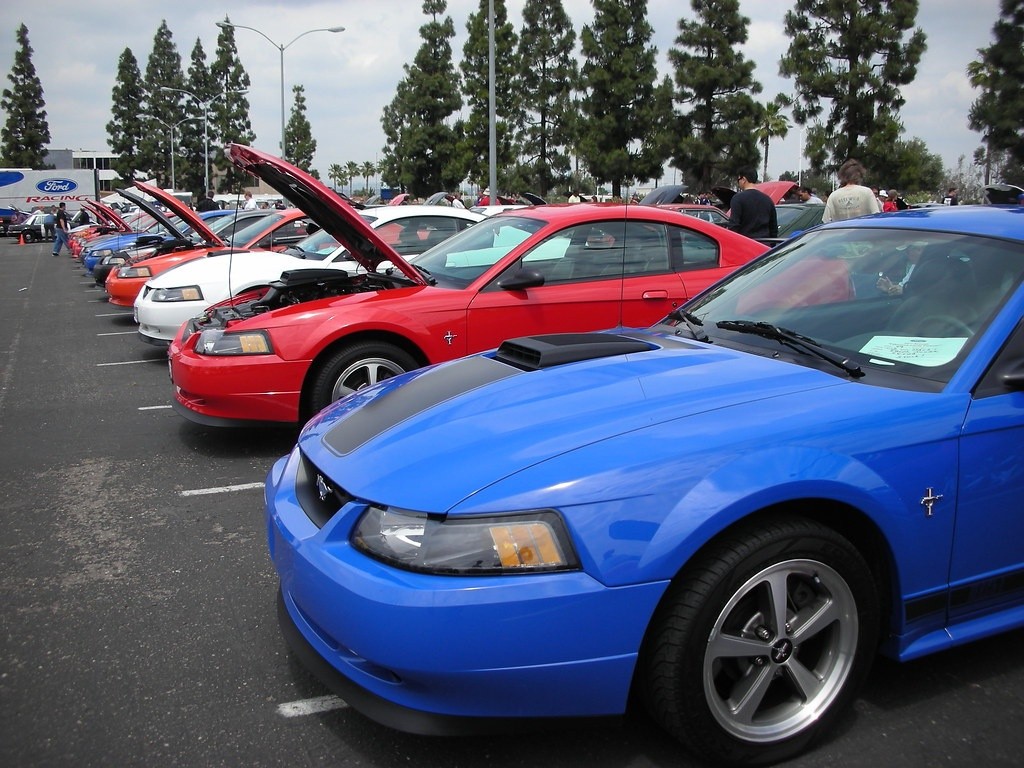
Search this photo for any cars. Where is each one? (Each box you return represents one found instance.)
[265,200,1024,768]
[167,141,857,439]
[133,204,589,347]
[6,209,97,243]
[66,187,309,289]
[104,179,432,307]
[361,182,1024,246]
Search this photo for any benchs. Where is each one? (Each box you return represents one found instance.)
[640,244,716,270]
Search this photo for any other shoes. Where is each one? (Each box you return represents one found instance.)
[52,252,61,256]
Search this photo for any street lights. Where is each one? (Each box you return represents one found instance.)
[137,114,205,190]
[160,87,250,198]
[215,21,346,207]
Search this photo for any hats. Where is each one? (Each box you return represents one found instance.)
[878,190,888,197]
[483,188,490,195]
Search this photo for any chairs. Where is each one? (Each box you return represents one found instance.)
[426,230,448,249]
[549,244,586,281]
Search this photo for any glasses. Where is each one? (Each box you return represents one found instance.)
[737,177,743,183]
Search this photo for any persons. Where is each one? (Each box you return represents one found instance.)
[52,202,71,256]
[13,211,25,222]
[822,159,881,267]
[871,241,927,298]
[51,205,58,213]
[681,191,712,221]
[44,213,55,242]
[452,193,466,209]
[727,166,778,247]
[630,194,641,204]
[31,205,45,214]
[567,190,580,203]
[474,187,500,206]
[783,185,831,204]
[401,196,409,205]
[195,191,220,212]
[869,185,910,212]
[244,191,286,210]
[78,208,89,225]
[941,187,958,206]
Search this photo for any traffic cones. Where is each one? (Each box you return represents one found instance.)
[19,234,27,247]
